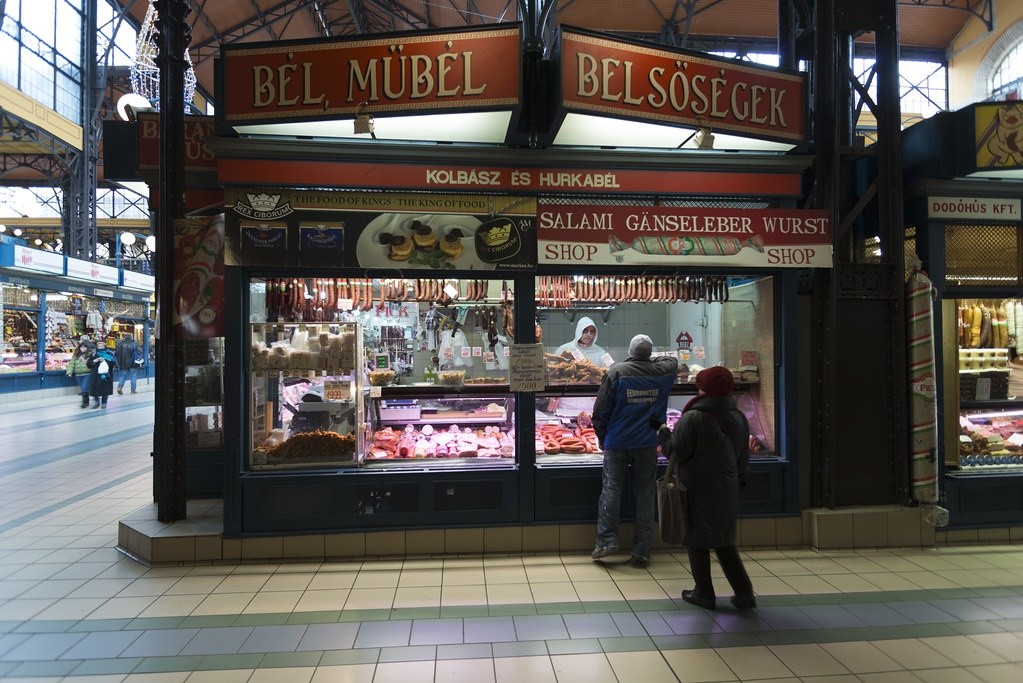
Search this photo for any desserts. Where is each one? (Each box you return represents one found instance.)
[382,220,465,261]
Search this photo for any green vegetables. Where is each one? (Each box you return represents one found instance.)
[407,248,473,270]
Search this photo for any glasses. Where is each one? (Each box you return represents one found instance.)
[583,328,595,336]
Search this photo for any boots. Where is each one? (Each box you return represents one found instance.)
[79,392,89,408]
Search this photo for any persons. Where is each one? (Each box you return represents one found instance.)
[590,334,678,562]
[66,340,93,409]
[86,340,116,409]
[115,334,141,395]
[553,317,615,410]
[659,365,757,609]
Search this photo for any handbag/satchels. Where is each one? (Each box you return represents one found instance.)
[656,450,687,545]
[97,360,109,375]
[129,341,145,368]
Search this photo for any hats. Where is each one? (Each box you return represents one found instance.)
[97,339,107,346]
[628,334,653,358]
[696,366,733,393]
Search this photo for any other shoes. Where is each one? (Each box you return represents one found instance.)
[92,404,100,409]
[591,544,619,559]
[631,549,650,567]
[131,390,137,394]
[118,387,123,395]
[731,595,756,609]
[682,590,716,610]
[101,405,107,409]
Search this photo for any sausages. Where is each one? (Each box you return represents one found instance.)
[175,273,200,316]
[539,276,729,308]
[265,277,513,338]
